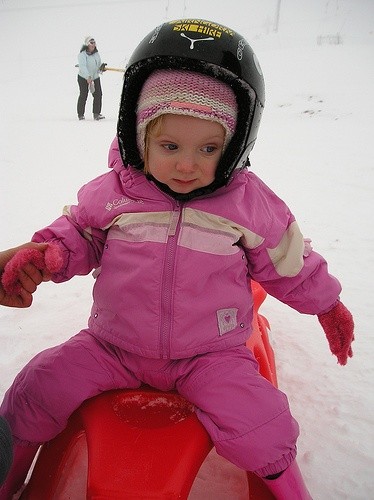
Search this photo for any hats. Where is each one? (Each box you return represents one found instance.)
[84,36,94,46]
[136,68,237,159]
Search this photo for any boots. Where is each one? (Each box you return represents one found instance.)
[259,454,313,500]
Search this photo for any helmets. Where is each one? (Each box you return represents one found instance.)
[115,18,265,185]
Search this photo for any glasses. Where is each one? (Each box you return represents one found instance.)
[89,41,95,45]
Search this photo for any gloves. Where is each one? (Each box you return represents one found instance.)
[2,244,65,299]
[318,300,356,367]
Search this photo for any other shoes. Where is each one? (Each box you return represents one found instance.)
[78,113,85,120]
[93,112,105,120]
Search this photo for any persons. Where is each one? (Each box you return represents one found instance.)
[0,19,354,500]
[77,37,107,120]
